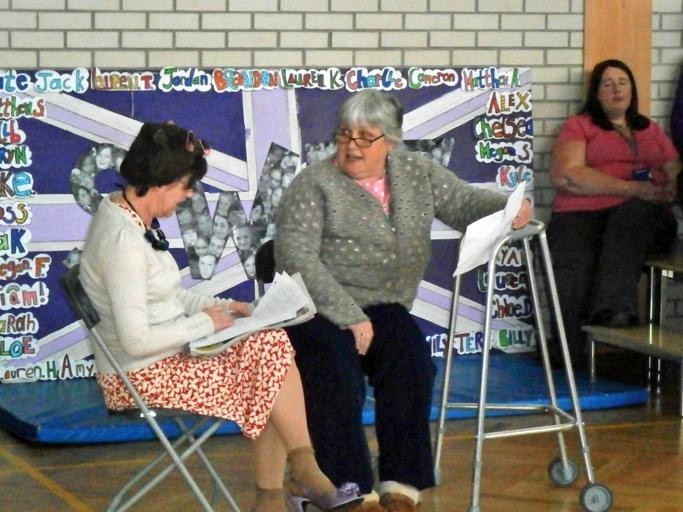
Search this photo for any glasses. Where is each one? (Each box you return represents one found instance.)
[331,129,384,150]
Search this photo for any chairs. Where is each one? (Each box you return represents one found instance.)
[59,264,246,512]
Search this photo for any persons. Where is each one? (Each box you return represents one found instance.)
[71,144,125,212]
[538,59,682,371]
[77,122,366,512]
[411,138,455,165]
[306,141,337,164]
[176,145,299,280]
[273,87,534,512]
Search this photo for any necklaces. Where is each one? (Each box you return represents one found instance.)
[608,117,640,157]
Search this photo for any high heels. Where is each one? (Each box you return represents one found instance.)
[285,476,364,512]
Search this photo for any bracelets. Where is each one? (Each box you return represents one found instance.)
[623,178,631,200]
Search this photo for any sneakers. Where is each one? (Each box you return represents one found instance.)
[378,480,422,512]
[347,487,379,512]
